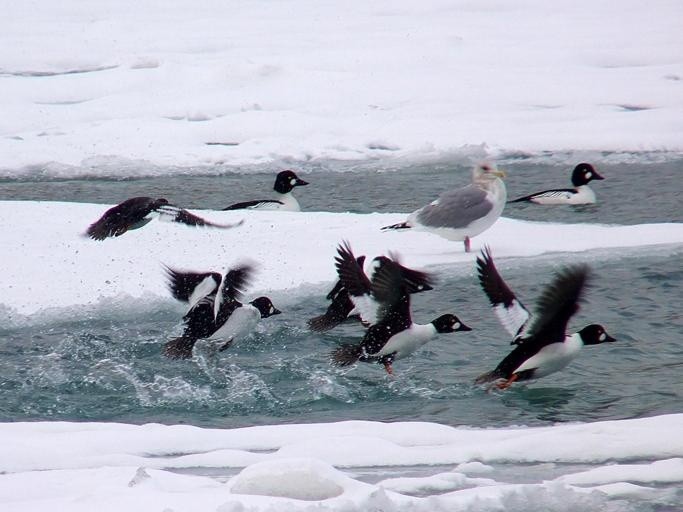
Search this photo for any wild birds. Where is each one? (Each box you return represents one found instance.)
[155,261,285,358]
[309,239,475,376]
[377,156,510,254]
[471,242,618,393]
[221,168,310,212]
[81,195,246,238]
[509,160,605,208]
